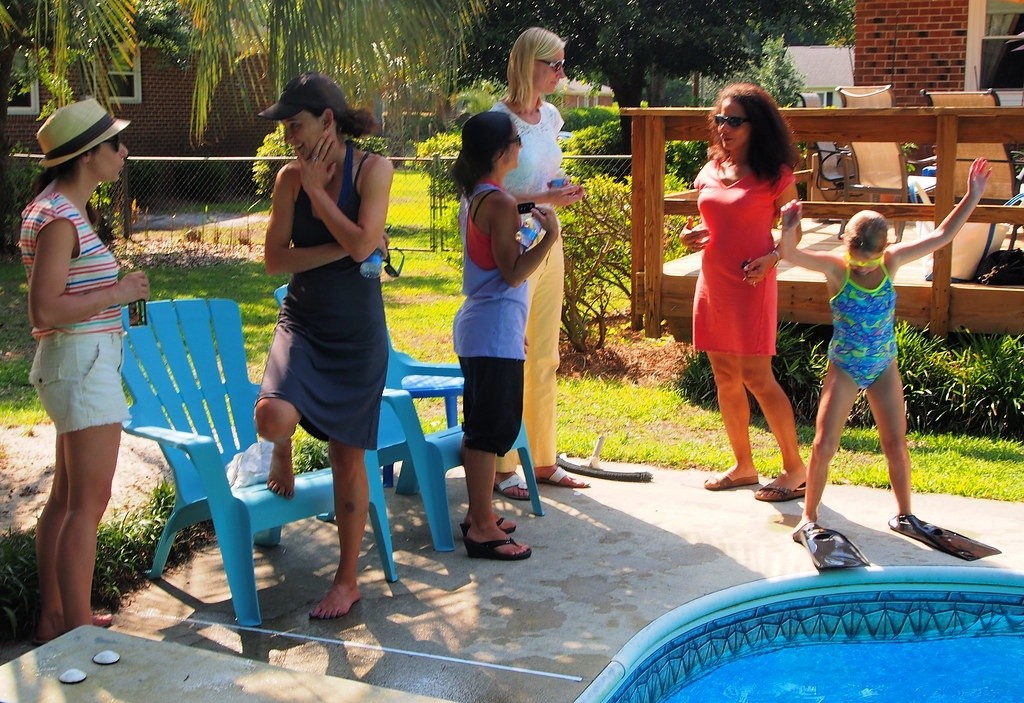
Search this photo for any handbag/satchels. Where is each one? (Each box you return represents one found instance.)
[975,247,1024,285]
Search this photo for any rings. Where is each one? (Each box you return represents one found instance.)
[753,281,755,285]
[310,157,318,160]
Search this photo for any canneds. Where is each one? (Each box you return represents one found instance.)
[128,300,148,328]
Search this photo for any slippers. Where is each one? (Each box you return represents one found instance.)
[536,466,589,488]
[755,482,806,501]
[33,607,113,644]
[494,474,530,500]
[704,472,759,490]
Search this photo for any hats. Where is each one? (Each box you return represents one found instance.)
[257,71,347,120]
[36,99,131,168]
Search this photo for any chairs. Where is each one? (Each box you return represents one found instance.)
[119,298,397,624]
[276,284,547,552]
[794,86,1024,287]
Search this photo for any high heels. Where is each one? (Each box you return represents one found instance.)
[460,518,516,537]
[463,537,532,561]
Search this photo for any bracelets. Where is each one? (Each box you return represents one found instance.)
[771,250,780,267]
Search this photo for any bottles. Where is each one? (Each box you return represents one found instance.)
[360,248,383,278]
[514,212,545,248]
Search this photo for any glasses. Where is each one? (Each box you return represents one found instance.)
[509,136,522,148]
[535,59,565,72]
[849,258,877,268]
[715,114,748,128]
[383,225,405,278]
[102,134,120,153]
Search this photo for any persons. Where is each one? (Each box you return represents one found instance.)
[253,71,395,620]
[452,111,558,560]
[20,99,151,643]
[679,83,807,503]
[780,158,1002,569]
[488,27,590,500]
